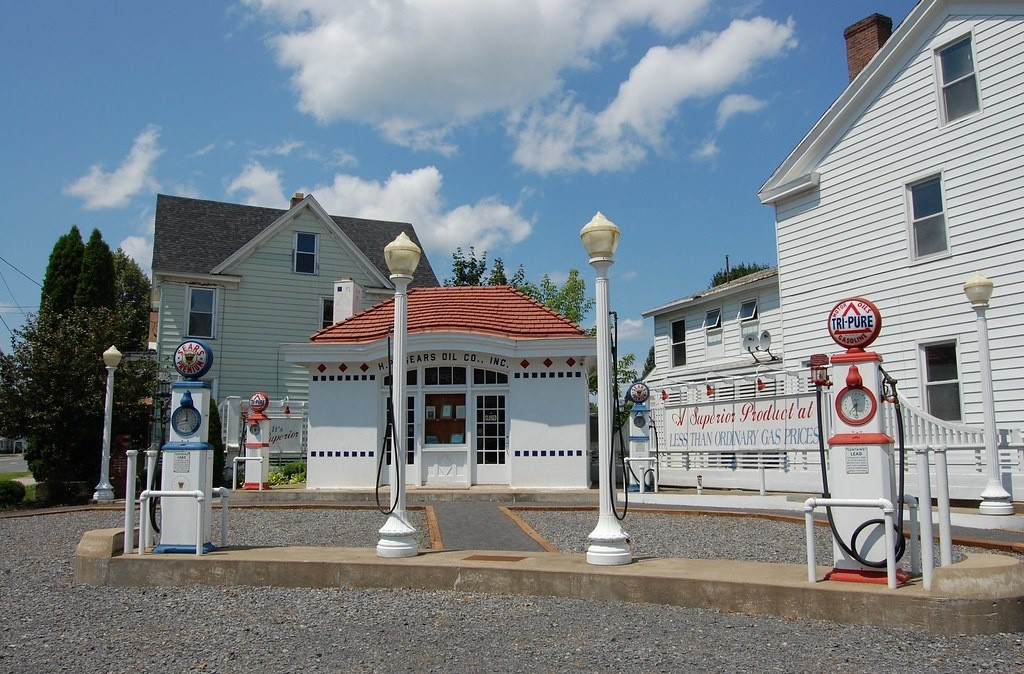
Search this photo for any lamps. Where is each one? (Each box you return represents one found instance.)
[281,396,291,414]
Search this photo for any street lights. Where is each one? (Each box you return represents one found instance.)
[579,210,635,567]
[376,229,424,558]
[92,343,123,501]
[961,269,1015,517]
